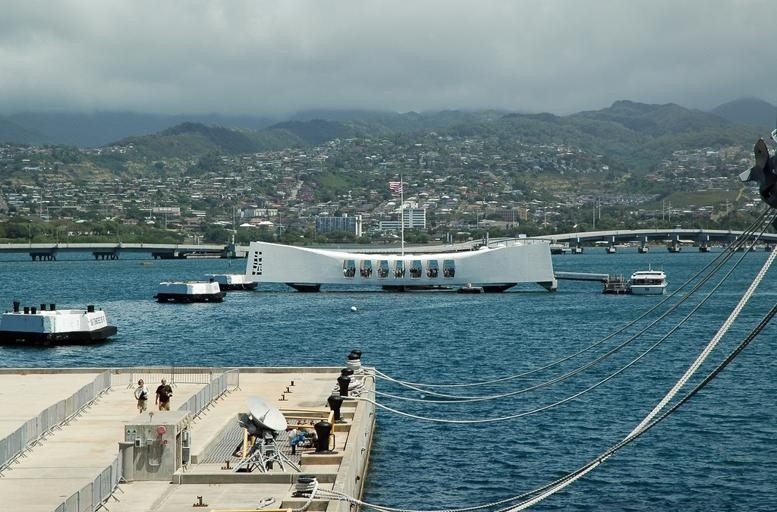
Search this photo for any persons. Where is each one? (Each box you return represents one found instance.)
[133,378,151,413]
[155,379,174,412]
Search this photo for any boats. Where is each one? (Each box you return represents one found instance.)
[1,349,377,509]
[205,272,257,290]
[239,238,558,295]
[152,280,226,303]
[628,263,667,295]
[602,275,629,294]
[0,300,117,350]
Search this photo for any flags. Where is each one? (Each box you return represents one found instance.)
[392,184,402,194]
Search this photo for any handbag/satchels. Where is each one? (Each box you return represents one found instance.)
[139,392,148,400]
